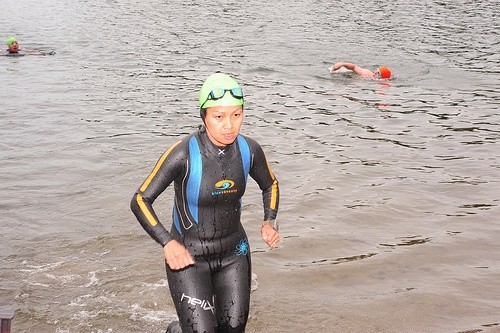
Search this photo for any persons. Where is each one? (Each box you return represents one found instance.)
[331,62,392,79]
[129,72,281,333]
[6,36,21,53]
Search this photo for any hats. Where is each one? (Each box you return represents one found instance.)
[199,73,243,109]
[379,67,391,79]
[7,37,17,46]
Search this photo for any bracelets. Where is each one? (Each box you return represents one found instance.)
[262,219,279,231]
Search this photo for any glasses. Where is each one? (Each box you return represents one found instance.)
[375,68,382,77]
[199,87,243,113]
